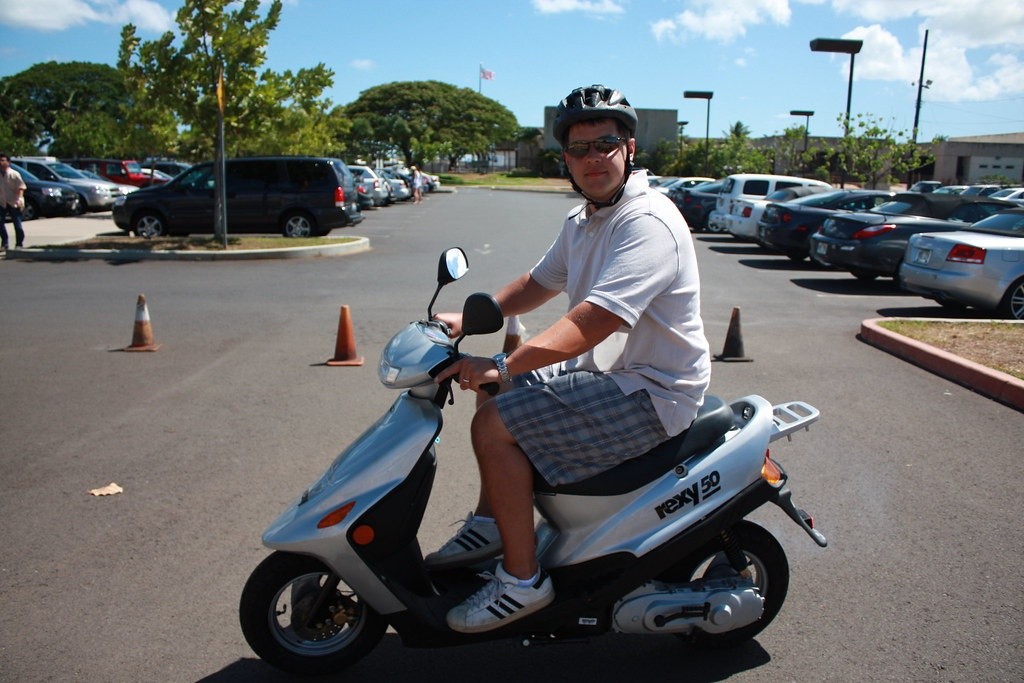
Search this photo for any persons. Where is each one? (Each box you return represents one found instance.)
[423,84,714,633]
[0,155,27,251]
[411,165,429,204]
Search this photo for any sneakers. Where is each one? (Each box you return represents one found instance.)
[447,562,554,633]
[425,511,540,564]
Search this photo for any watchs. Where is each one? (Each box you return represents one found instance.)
[491,353,512,385]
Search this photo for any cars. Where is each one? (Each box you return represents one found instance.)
[376,164,440,210]
[140,162,191,179]
[647,175,728,234]
[352,180,375,211]
[728,180,1024,322]
[6,155,140,222]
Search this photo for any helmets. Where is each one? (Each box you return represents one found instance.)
[552,85,638,146]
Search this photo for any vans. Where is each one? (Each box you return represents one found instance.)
[112,155,362,240]
[715,174,832,242]
[347,165,387,208]
[63,157,168,191]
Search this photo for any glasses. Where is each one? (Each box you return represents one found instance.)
[565,136,625,157]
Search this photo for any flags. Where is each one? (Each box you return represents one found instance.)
[480,67,493,81]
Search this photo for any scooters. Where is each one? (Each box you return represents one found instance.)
[239,247,826,674]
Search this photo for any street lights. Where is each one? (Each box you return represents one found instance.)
[810,37,862,189]
[684,90,713,176]
[790,111,814,178]
[677,121,688,164]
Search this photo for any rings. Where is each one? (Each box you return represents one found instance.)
[462,378,470,383]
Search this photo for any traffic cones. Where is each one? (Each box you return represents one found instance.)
[326,306,365,366]
[123,293,162,350]
[501,313,523,356]
[714,306,754,363]
